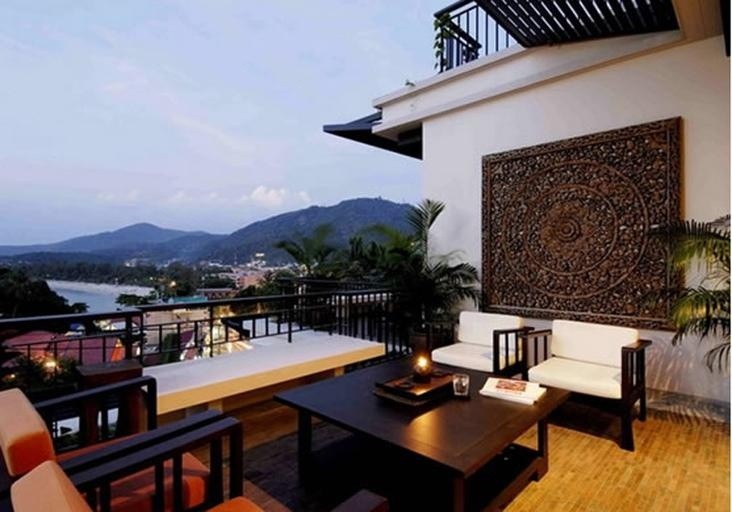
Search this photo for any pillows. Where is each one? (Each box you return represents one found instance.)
[550,318,639,365]
[0,390,57,477]
[458,311,522,349]
[9,461,92,512]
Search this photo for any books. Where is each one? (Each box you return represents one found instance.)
[480,376,547,406]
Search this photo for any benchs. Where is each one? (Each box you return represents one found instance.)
[140,330,386,465]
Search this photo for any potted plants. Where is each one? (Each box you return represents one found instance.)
[371,199,485,352]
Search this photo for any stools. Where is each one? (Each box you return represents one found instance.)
[72,360,142,447]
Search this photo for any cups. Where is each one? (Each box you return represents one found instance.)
[452,373,470,396]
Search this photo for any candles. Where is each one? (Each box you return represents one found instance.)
[413,353,432,383]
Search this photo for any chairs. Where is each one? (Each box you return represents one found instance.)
[520,331,652,452]
[0,375,225,512]
[431,310,534,381]
[8,416,267,512]
